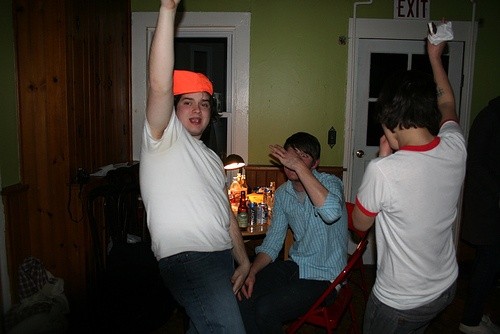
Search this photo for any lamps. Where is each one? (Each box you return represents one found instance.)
[223,154,245,187]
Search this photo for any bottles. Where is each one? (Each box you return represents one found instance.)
[230,195,239,212]
[263,188,273,210]
[245,196,251,210]
[237,190,248,231]
[228,172,248,199]
[251,202,268,226]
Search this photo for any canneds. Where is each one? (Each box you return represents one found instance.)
[263,188,272,205]
[247,202,268,224]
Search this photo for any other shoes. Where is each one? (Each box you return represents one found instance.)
[458,314,500,334]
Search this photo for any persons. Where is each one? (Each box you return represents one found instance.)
[138,0,251,334]
[459,96,500,334]
[353,32,467,334]
[223,154,252,194]
[234,133,348,334]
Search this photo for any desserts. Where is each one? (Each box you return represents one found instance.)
[427,21,437,35]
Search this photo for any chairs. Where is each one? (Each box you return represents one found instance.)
[67,183,157,334]
[284,202,369,334]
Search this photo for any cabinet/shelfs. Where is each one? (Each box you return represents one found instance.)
[11,0,131,300]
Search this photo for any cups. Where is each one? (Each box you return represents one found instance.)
[270,182,275,192]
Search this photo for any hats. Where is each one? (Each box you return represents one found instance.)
[173,70,214,97]
